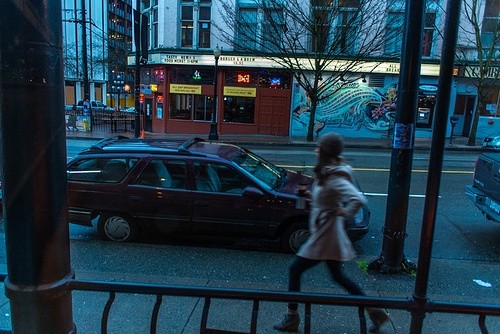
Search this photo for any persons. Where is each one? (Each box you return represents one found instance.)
[272,132,396,334]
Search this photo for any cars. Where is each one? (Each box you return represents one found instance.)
[464,151,500,226]
[482,134,500,151]
[123,102,163,119]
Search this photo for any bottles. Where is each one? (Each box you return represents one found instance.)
[296,181,308,209]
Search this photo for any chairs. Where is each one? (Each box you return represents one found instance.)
[148,159,180,187]
[196,162,242,193]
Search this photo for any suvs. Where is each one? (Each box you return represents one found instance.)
[65,133,371,254]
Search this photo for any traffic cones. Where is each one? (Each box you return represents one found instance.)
[141,129,146,139]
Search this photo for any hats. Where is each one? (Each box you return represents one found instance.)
[319,132,344,156]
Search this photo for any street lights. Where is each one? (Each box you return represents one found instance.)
[208,44,222,140]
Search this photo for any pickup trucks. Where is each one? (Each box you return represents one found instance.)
[65,100,114,112]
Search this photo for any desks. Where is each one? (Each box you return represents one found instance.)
[101,117,135,132]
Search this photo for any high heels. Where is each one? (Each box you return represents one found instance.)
[368,309,397,334]
[273,312,301,333]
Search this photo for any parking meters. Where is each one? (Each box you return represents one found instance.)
[449,115,460,145]
